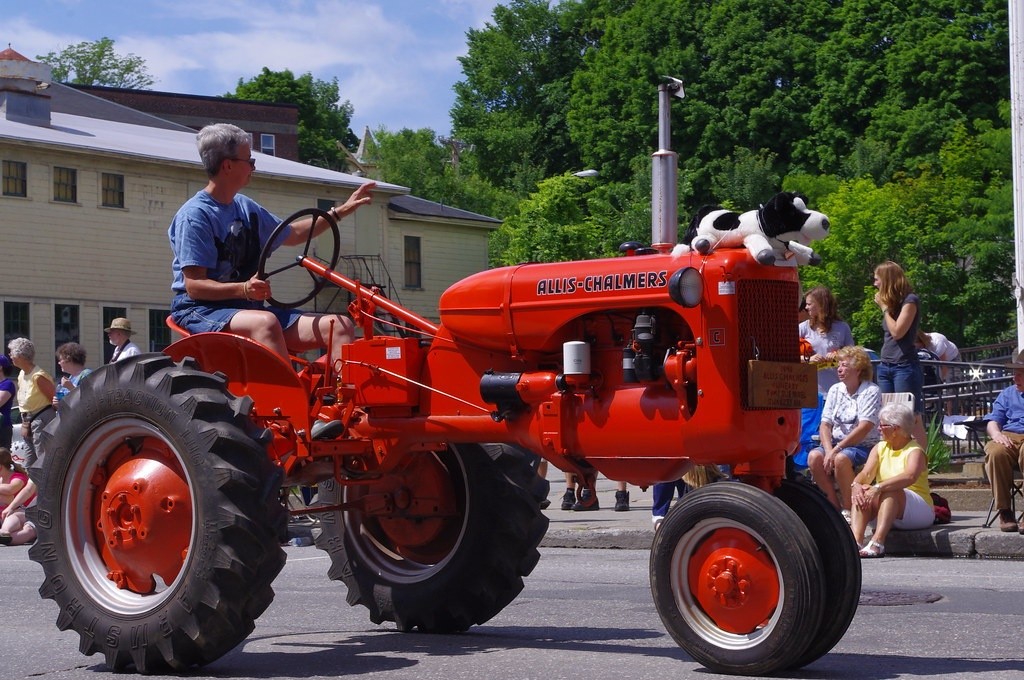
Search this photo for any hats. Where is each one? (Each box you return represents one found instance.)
[105,318,137,335]
[1005,349,1023,369]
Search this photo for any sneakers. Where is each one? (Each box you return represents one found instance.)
[558,488,576,511]
[614,491,630,511]
[571,489,599,511]
[540,499,551,510]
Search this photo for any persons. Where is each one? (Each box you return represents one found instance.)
[530,261,964,558]
[104,318,140,363]
[168,123,378,439]
[984,349,1024,534]
[0,337,93,545]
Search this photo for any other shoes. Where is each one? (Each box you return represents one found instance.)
[0,532,12,544]
[309,419,345,439]
[1000,508,1018,531]
[841,510,852,525]
[651,515,664,524]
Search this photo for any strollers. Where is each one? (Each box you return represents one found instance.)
[918,348,943,430]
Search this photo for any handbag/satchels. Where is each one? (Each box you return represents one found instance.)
[929,492,951,524]
[20,420,31,437]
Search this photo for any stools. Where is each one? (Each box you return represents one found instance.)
[981,468,1024,528]
[166,313,194,337]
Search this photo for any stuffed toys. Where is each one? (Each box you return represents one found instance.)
[670,190,832,266]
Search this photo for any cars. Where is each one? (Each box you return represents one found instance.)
[10,406,25,442]
[863,347,881,383]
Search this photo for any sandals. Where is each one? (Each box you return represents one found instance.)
[858,541,886,558]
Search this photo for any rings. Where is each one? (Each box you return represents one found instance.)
[264,292,268,299]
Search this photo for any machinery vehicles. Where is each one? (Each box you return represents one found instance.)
[24,74,864,677]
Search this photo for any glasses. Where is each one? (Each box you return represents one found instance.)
[834,402,858,425]
[878,423,893,429]
[230,158,256,168]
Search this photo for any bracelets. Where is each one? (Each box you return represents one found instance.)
[332,206,342,222]
[835,443,843,450]
[875,484,882,492]
[244,281,256,303]
[883,306,888,315]
[850,481,859,488]
